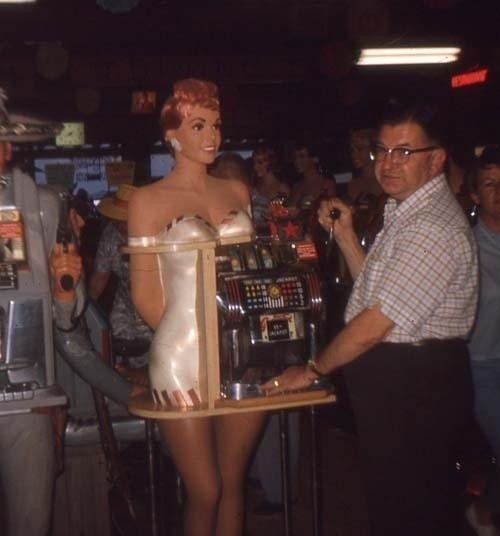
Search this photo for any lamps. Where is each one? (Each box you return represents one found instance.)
[354,46,460,67]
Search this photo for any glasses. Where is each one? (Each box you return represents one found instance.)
[368,145,437,165]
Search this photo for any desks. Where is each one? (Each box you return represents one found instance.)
[128,390,337,536]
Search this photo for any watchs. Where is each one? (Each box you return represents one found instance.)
[307,359,324,377]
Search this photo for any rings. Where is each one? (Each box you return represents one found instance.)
[275,380,279,387]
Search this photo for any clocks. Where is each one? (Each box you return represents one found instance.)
[50,120,88,146]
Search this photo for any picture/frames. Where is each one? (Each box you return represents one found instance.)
[129,88,159,115]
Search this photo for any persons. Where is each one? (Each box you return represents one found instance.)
[128,81,267,534]
[2,106,65,535]
[210,152,252,191]
[249,141,290,233]
[463,153,500,535]
[345,128,385,256]
[88,183,155,383]
[260,103,479,536]
[288,138,336,208]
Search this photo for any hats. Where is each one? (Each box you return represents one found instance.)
[96,184,139,220]
